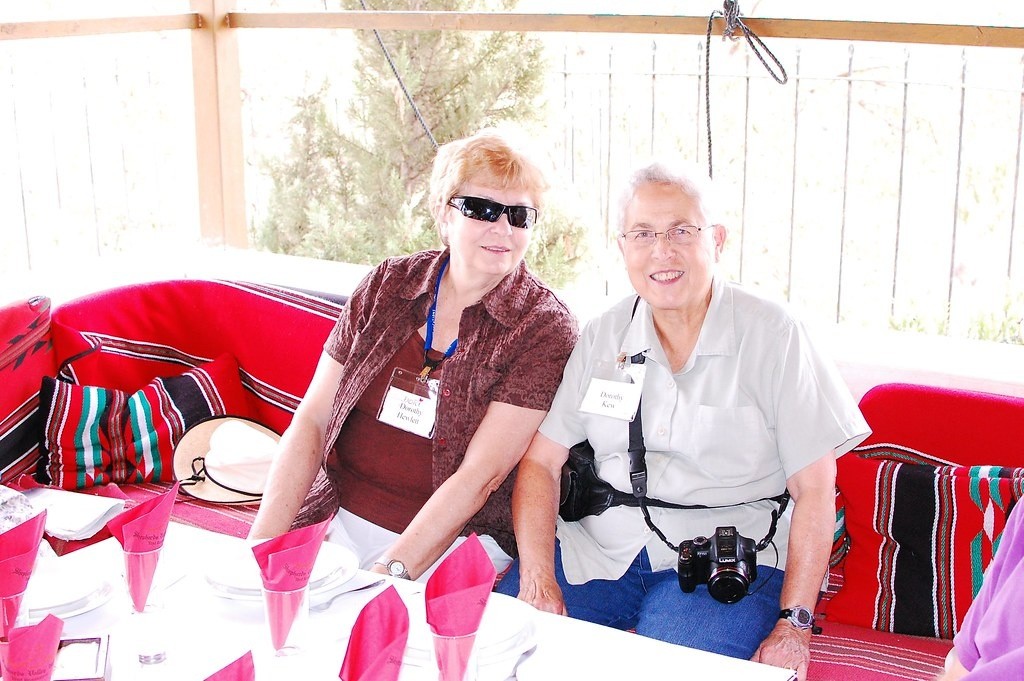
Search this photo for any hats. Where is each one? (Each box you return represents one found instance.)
[172,414,287,504]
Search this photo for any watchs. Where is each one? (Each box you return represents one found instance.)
[779,605,823,634]
[375,556,411,580]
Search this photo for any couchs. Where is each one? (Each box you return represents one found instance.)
[0,275,1024,681]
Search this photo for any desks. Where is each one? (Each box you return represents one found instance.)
[0,478,799,681]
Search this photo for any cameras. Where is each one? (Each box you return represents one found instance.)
[677,524,758,602]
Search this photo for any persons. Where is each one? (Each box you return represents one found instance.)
[245,130,580,582]
[490,158,874,680]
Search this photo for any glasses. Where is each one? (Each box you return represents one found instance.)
[620,225,715,245]
[447,195,539,229]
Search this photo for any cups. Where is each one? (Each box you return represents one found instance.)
[428,620,482,681]
[0,583,33,644]
[118,544,170,626]
[257,582,314,667]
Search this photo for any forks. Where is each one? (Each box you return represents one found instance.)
[309,578,386,613]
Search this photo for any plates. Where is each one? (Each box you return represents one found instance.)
[198,534,361,601]
[402,589,531,650]
